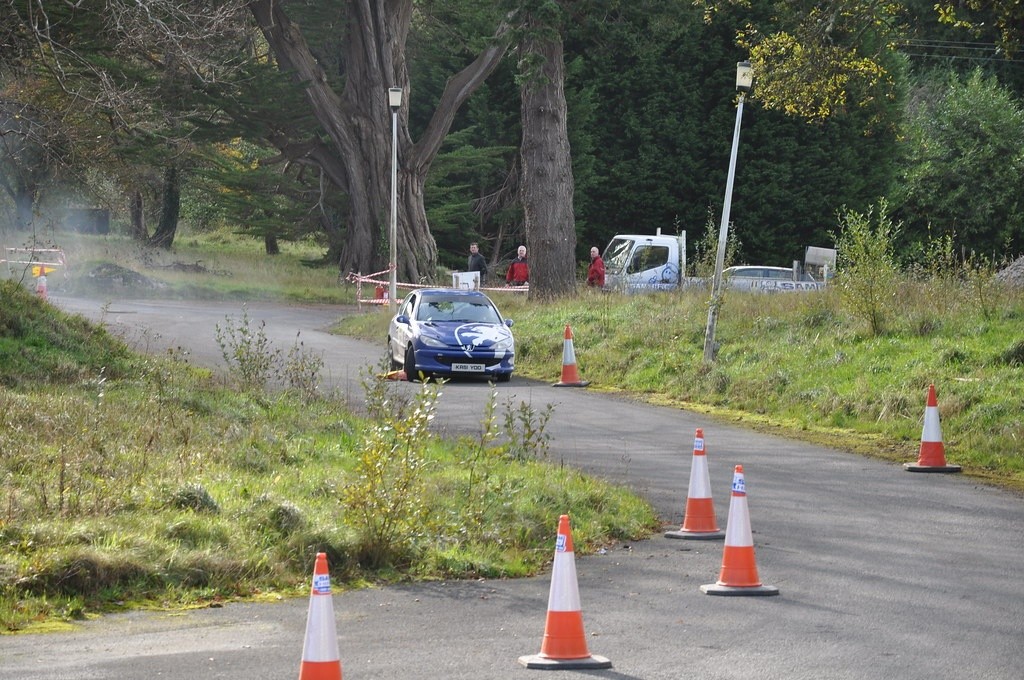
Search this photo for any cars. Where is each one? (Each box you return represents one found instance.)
[386,288,516,383]
[709,265,818,280]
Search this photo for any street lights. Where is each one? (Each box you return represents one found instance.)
[386,83,404,307]
[702,62,760,360]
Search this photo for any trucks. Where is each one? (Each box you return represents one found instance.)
[596,226,828,293]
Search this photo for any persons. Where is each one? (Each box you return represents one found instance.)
[588,247,605,289]
[505,245,529,288]
[466,241,491,287]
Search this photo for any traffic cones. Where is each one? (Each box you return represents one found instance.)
[902,382,965,472]
[377,369,402,380]
[552,323,592,388]
[698,463,780,596]
[35,262,49,301]
[663,427,728,537]
[295,552,347,680]
[518,514,615,671]
